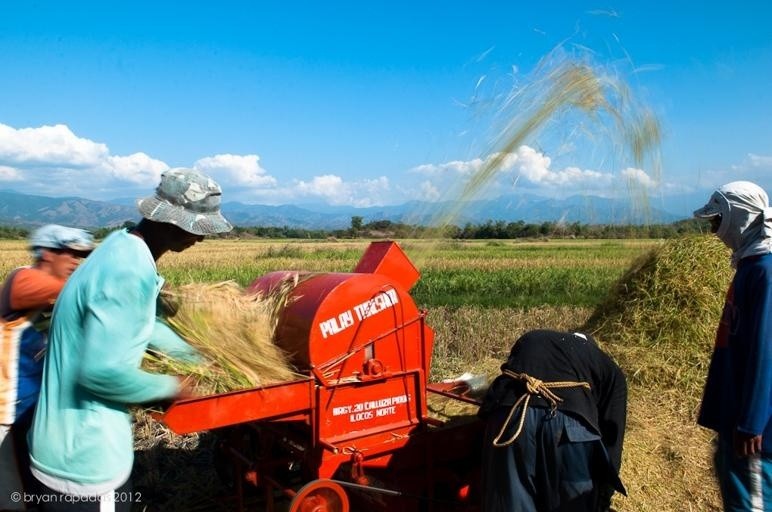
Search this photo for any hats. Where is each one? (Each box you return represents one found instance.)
[30,223,96,258]
[137,168,233,236]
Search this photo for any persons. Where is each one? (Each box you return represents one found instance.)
[25,166,233,511]
[477,328,628,511]
[0,224,98,512]
[695,180,772,512]
